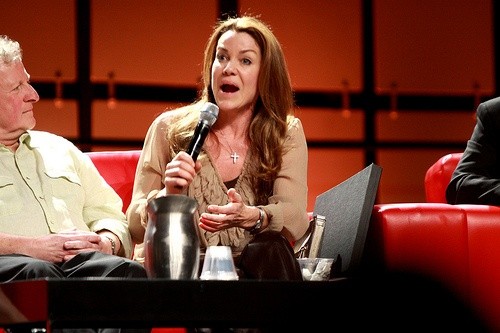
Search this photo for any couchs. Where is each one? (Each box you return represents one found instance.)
[0,150,500,333]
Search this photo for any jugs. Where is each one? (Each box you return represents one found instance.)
[144,194,202,279]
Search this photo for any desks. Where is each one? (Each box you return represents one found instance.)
[0,277,349,333]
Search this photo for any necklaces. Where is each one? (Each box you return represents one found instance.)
[218,123,246,164]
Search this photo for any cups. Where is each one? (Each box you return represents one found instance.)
[297,258,335,280]
[200,245,239,281]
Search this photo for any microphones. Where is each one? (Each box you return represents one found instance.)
[174,102,219,190]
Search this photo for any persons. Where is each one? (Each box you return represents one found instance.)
[0,36,147,280]
[446,95,500,206]
[124,17,309,283]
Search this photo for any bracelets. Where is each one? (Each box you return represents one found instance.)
[105,237,117,255]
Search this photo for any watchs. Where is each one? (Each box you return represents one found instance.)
[250,207,265,233]
